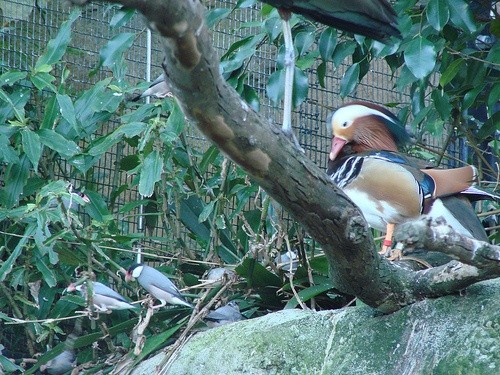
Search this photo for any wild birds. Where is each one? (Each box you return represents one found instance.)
[125,72,174,100]
[202,267,239,285]
[67,280,137,321]
[323,100,500,262]
[125,263,198,310]
[274,249,300,275]
[203,300,241,328]
[39,334,79,375]
